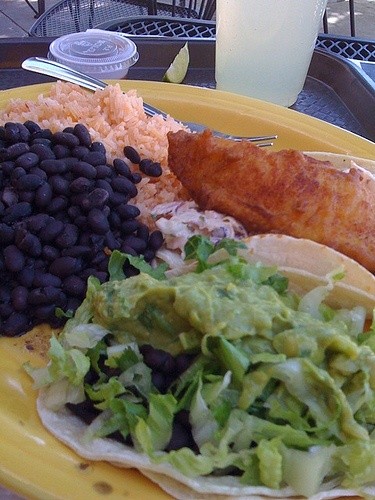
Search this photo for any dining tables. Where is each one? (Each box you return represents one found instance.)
[0,37,375,140]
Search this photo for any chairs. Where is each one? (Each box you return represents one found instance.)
[0,0,375,60]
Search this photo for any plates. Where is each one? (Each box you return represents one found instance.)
[1,79,374,499]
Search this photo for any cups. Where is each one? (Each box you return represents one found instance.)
[48,31,139,78]
[215,0,328,108]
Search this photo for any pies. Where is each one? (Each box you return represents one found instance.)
[152,150,375,298]
[32,267,375,500]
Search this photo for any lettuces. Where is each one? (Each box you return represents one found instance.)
[20,225,374,500]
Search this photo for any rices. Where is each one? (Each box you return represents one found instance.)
[0,77,198,226]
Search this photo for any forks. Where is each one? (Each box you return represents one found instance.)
[21,56,276,148]
